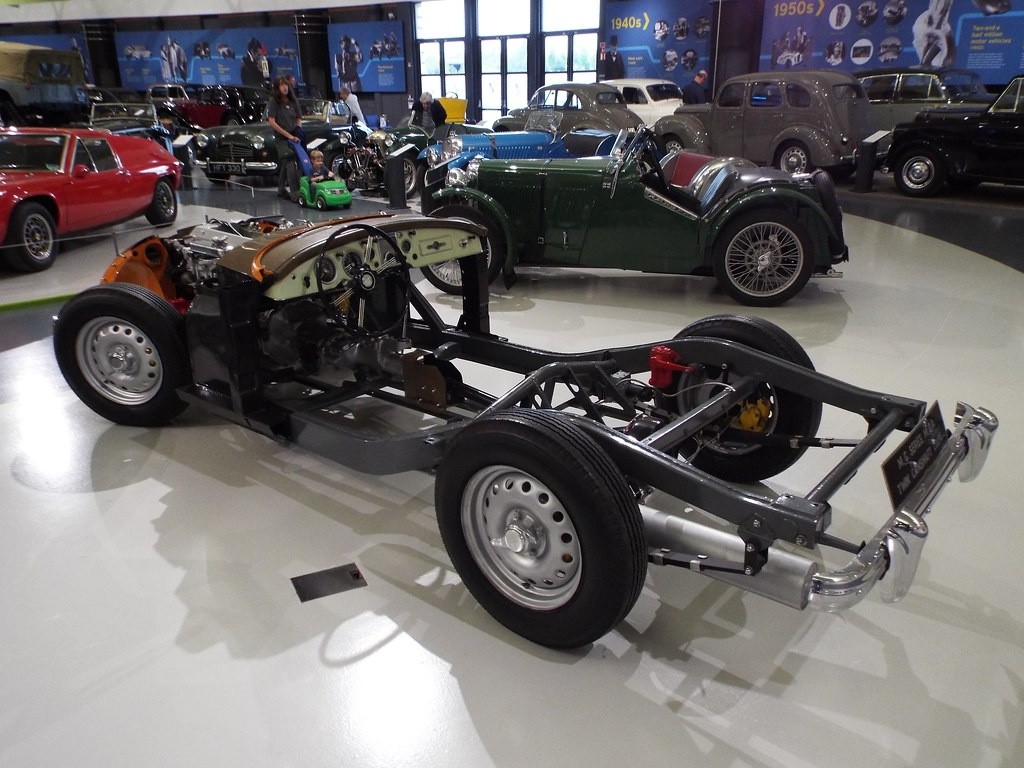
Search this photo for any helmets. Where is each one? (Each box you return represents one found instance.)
[339,35,350,43]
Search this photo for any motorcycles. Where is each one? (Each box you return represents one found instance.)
[327,131,418,200]
[53,216,999,652]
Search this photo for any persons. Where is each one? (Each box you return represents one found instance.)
[603,35,625,78]
[682,69,708,103]
[267,77,302,205]
[411,92,448,128]
[892,1,906,21]
[335,35,364,93]
[828,47,842,65]
[339,88,366,126]
[286,73,297,90]
[240,37,267,86]
[160,36,187,83]
[298,150,335,202]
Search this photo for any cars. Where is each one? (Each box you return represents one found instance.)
[0,40,353,272]
[492,83,645,132]
[855,68,995,169]
[655,69,891,173]
[420,125,849,307]
[333,120,493,200]
[299,175,352,210]
[882,76,1024,198]
[600,79,683,131]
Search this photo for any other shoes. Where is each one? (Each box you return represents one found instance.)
[277,190,291,199]
[290,193,299,203]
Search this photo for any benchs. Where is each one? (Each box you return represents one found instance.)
[305,114,348,126]
[670,164,738,209]
